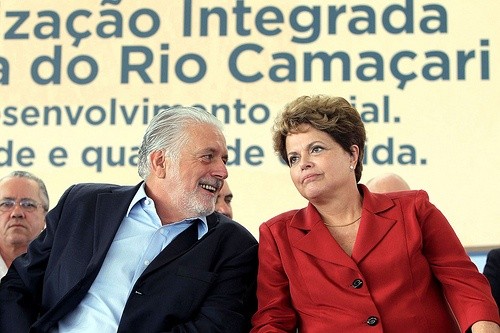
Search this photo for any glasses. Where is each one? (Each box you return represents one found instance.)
[0,199,42,212]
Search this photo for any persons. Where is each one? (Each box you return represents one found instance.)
[366,173,411,194]
[253,95,500,333]
[0,171,49,279]
[0,107,259,333]
[215,180,233,219]
